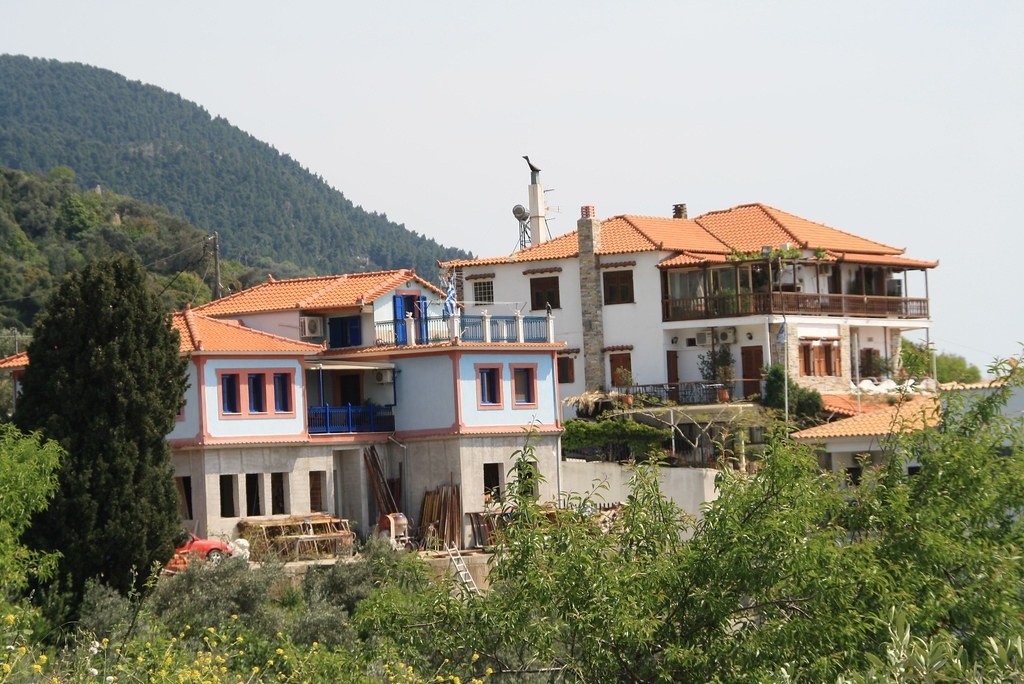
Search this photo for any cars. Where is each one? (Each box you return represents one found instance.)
[160,527,232,576]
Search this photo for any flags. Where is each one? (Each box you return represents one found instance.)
[442,277,458,321]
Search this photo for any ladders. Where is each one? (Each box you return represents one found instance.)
[443,540,480,598]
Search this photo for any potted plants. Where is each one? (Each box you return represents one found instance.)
[615,366,633,405]
[715,365,735,402]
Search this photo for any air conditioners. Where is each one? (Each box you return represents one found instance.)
[299,315,323,338]
[718,327,737,344]
[373,370,396,384]
[695,330,712,346]
[819,264,833,278]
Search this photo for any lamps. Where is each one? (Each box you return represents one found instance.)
[761,245,773,256]
